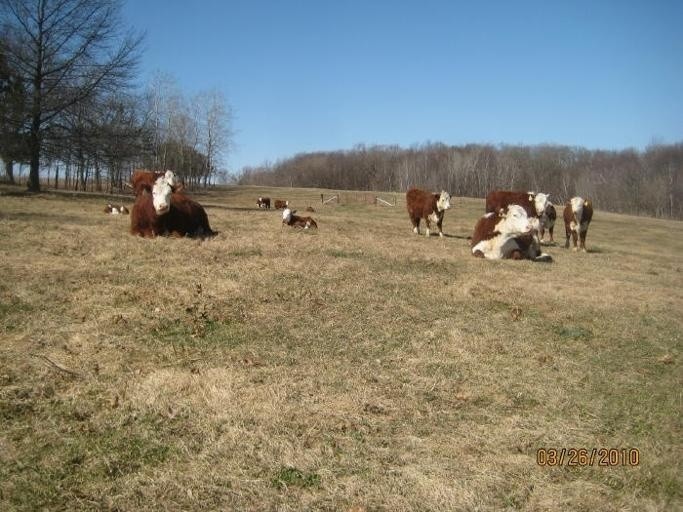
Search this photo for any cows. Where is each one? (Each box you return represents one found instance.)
[563,196,593,253]
[255,196,318,231]
[469,188,557,263]
[406,188,452,238]
[103,169,221,242]
